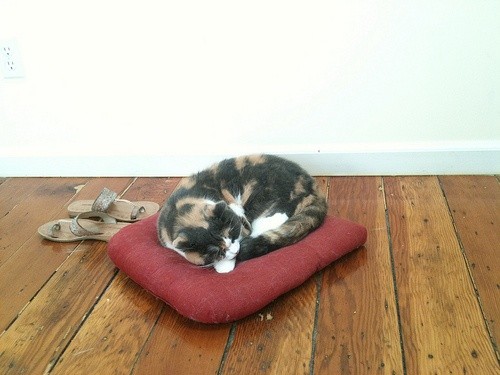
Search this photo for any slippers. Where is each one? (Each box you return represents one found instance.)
[37,187,160,243]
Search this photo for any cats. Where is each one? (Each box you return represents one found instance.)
[156,154,327,274]
